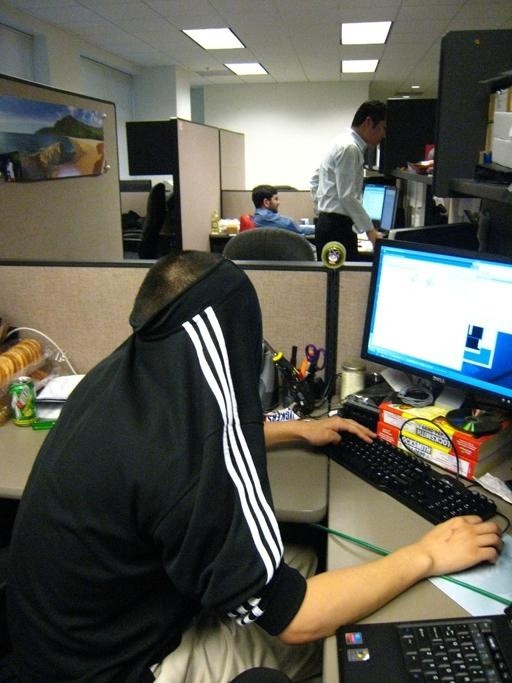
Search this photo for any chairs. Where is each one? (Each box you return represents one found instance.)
[221,228,315,259]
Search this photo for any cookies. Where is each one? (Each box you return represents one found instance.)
[0,338,48,422]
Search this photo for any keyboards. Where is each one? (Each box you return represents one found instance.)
[321,431,497,525]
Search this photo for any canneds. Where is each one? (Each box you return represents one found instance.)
[8,376,37,426]
[340,360,384,402]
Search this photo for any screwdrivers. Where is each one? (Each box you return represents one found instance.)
[262,337,297,380]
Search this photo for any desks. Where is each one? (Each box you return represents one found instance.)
[0,369,329,524]
[323,392,512,683]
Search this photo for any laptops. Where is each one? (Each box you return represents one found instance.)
[335,603,512,682]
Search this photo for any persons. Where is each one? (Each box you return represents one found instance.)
[0,250,505,682]
[138,182,168,258]
[252,100,389,262]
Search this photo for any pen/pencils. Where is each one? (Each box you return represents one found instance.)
[290,346,315,383]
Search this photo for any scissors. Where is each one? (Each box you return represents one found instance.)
[306,344,327,381]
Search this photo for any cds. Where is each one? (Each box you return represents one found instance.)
[445,406,501,435]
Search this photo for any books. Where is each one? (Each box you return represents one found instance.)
[377,381,512,482]
[36,374,87,421]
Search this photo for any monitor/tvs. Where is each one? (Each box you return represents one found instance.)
[360,238,512,416]
[120,180,151,194]
[119,190,152,226]
[379,186,400,238]
[362,182,386,223]
[388,223,477,251]
